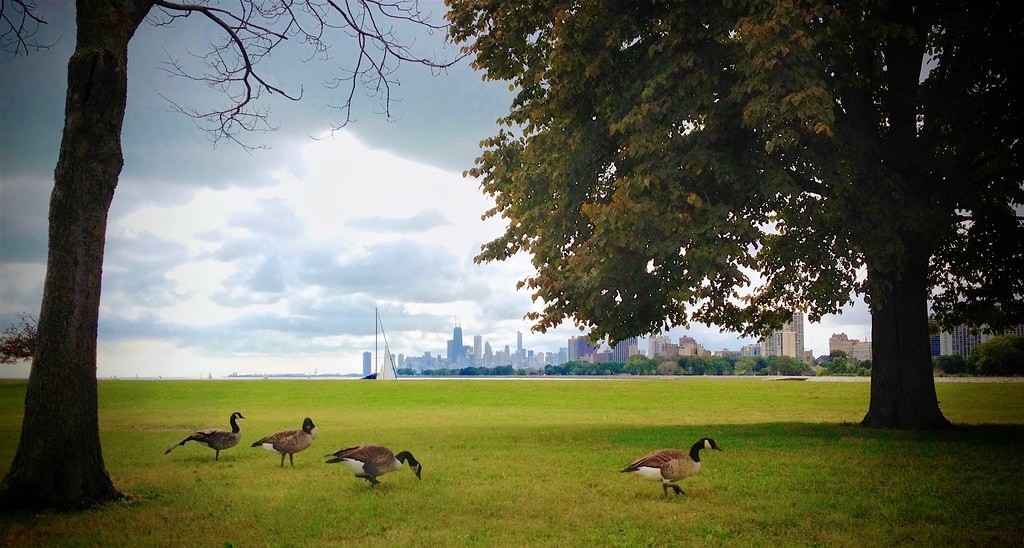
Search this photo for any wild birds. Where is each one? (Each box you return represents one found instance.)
[250,417,319,468]
[323,443,422,488]
[620,438,723,499]
[165,412,245,461]
